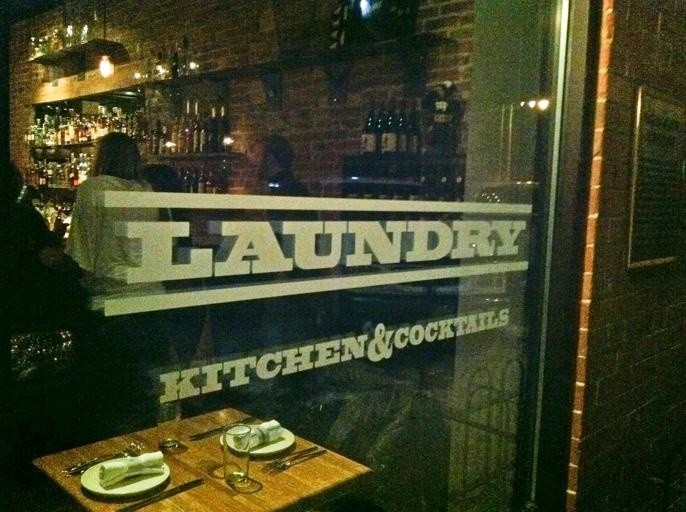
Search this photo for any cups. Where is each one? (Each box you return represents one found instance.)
[156,397,184,453]
[222,424,251,485]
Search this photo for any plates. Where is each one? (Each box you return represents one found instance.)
[219,423,296,458]
[80,456,171,499]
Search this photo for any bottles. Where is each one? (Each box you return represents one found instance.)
[33,197,75,239]
[25,98,235,154]
[128,35,205,83]
[21,153,97,190]
[359,98,451,155]
[146,159,235,195]
[26,23,91,62]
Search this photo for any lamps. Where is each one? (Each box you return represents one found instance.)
[97,4,116,76]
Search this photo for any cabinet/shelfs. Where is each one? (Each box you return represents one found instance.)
[21,28,247,214]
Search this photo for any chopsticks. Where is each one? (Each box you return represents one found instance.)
[188,414,259,442]
[114,479,204,511]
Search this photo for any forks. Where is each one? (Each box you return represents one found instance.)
[260,445,328,475]
[62,440,144,478]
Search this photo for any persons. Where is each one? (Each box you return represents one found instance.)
[10,133,177,312]
[252,135,319,250]
